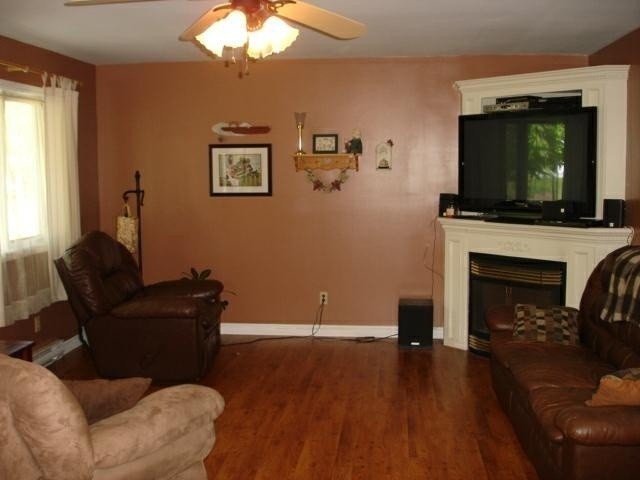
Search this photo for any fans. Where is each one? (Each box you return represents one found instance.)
[64,0,368,42]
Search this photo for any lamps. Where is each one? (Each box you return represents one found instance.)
[193,8,298,65]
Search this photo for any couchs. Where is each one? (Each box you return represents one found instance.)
[53,228,225,386]
[486,245,640,479]
[2,352,224,479]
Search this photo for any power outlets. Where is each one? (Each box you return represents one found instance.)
[319,292,328,305]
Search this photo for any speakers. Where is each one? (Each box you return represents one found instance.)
[439,193,458,216]
[398,298,434,345]
[602,199,625,227]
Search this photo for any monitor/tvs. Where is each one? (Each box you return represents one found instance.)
[458,107,598,224]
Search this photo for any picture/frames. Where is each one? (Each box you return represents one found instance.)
[208,144,273,197]
[311,133,339,155]
